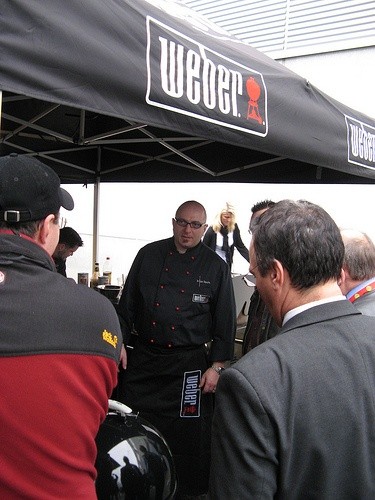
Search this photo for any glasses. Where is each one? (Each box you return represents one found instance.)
[242,266,262,287]
[174,217,207,230]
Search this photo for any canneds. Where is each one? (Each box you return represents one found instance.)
[98,276,109,286]
[78,273,88,287]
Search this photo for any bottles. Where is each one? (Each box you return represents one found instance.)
[91,263,101,290]
[103,257,111,285]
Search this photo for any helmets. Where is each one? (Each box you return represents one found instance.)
[94,400,178,500]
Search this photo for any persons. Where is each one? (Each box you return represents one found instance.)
[0,152,122,500]
[118,200,236,500]
[52,227,83,278]
[203,200,375,500]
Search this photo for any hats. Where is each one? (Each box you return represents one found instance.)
[0,151,76,225]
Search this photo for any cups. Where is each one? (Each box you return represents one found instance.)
[78,273,88,287]
[98,276,109,284]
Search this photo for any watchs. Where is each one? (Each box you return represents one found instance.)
[210,365,225,375]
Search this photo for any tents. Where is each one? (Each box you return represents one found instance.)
[0,0,375,281]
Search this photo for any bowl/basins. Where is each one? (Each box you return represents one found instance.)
[94,285,121,298]
